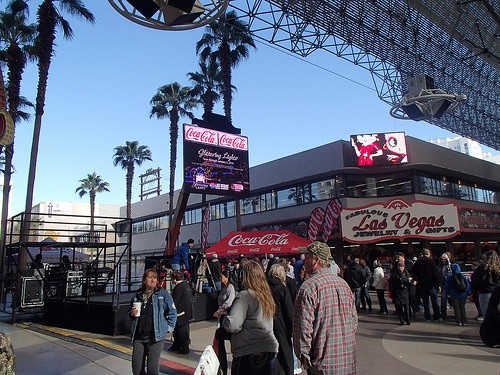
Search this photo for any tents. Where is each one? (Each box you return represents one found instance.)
[203,231,315,260]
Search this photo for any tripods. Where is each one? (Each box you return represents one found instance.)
[196,259,217,293]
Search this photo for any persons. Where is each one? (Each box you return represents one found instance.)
[220,262,279,375]
[169,239,194,273]
[351,134,406,167]
[149,252,208,294]
[388,249,500,348]
[168,272,193,354]
[29,254,44,277]
[292,241,358,375]
[54,256,71,275]
[128,270,177,375]
[210,253,373,374]
[265,264,294,375]
[371,260,389,314]
[214,270,236,328]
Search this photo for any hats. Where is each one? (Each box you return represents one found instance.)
[307,241,331,262]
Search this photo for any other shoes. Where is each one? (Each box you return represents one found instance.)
[356,308,486,327]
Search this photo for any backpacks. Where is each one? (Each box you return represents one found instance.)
[452,273,467,294]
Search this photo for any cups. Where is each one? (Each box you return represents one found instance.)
[133,302,142,317]
